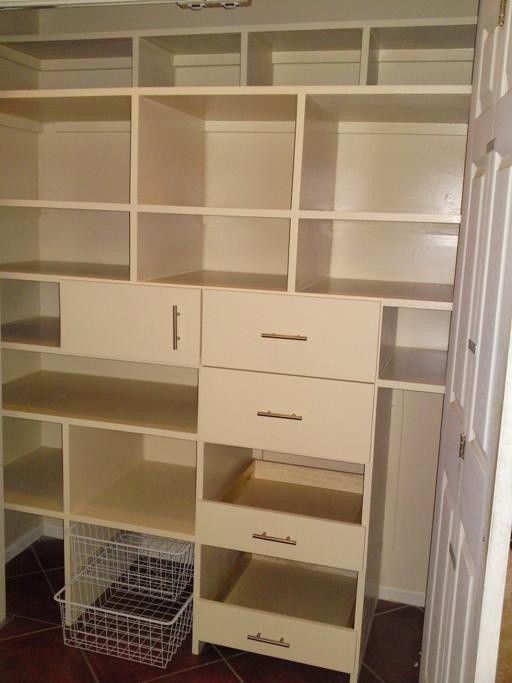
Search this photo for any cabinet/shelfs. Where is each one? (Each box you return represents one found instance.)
[0,0,475,683]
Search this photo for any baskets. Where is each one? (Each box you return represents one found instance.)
[64,521,194,603]
[53,578,194,670]
